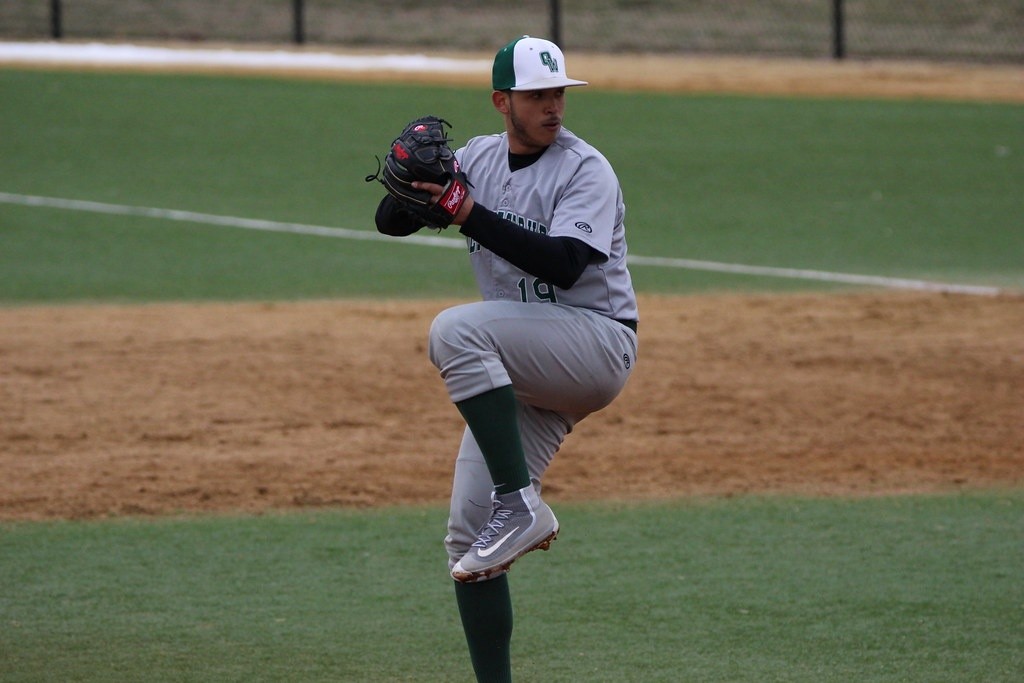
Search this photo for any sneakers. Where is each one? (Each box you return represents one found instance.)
[449,477,559,583]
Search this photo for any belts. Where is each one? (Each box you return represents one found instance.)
[615,316,637,333]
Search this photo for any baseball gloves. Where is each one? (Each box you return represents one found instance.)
[363,115,477,235]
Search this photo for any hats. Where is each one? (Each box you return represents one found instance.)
[492,35,586,90]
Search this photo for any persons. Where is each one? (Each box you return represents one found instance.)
[376,35,640,683]
